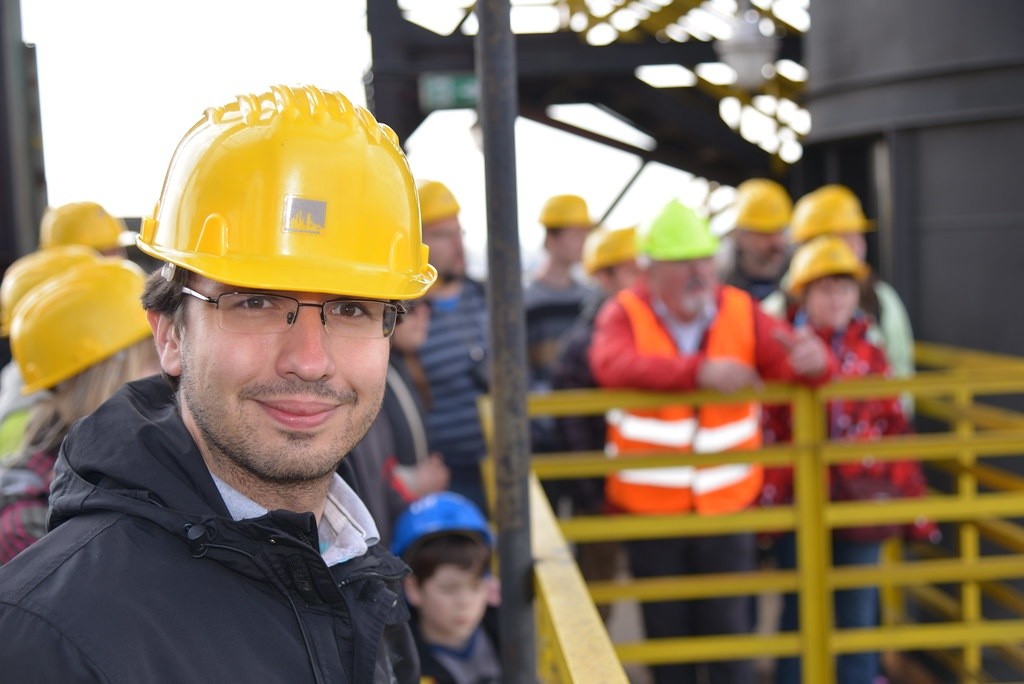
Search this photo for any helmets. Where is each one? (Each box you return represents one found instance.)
[9,256,155,394]
[539,194,595,229]
[732,178,792,235]
[36,202,139,252]
[388,493,496,578]
[0,244,104,341]
[644,198,719,262]
[788,183,877,246]
[585,226,637,275]
[787,234,869,300]
[414,178,459,227]
[132,85,439,302]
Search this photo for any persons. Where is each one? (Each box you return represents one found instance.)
[403,173,532,524]
[383,488,506,684]
[757,183,920,424]
[586,201,840,683]
[373,302,453,505]
[753,236,942,684]
[40,208,143,263]
[519,195,609,514]
[3,74,440,684]
[0,241,107,465]
[709,178,793,301]
[549,228,651,627]
[0,254,163,574]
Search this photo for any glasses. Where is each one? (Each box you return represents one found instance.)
[182,285,396,334]
